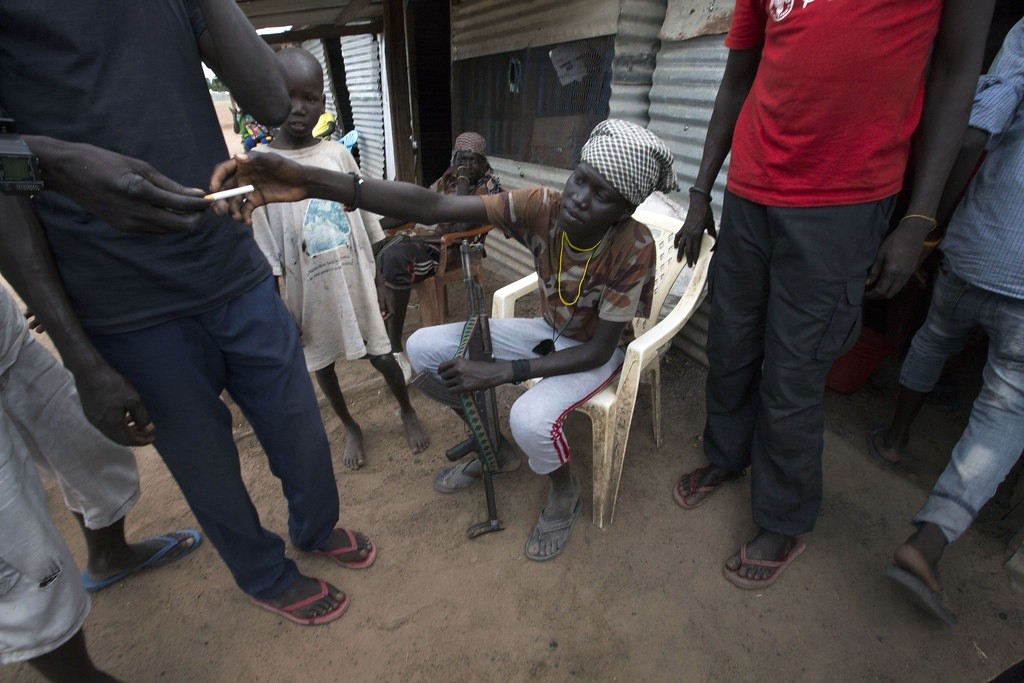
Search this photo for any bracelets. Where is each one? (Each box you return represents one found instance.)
[457,176,470,183]
[344,171,363,212]
[901,214,937,233]
[511,358,529,385]
[689,187,712,203]
[923,239,940,247]
[457,166,467,172]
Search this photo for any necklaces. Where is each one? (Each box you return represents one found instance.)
[531,231,602,356]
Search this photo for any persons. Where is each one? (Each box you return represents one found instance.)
[251,49,431,471]
[0,1,376,624]
[209,119,680,560]
[0,282,202,683]
[870,0,1024,625]
[229,107,273,153]
[380,132,502,356]
[673,0,996,590]
[312,103,335,141]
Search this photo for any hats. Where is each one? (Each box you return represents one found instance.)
[454,131,486,155]
[579,119,679,206]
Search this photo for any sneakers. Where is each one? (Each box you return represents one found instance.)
[392,352,411,385]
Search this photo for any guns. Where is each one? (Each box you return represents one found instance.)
[407,235,506,540]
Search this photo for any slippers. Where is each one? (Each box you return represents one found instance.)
[674,462,732,509]
[323,527,378,568]
[724,536,806,589]
[881,568,957,624]
[247,576,350,625]
[866,421,901,468]
[526,495,583,559]
[435,458,509,494]
[81,529,201,590]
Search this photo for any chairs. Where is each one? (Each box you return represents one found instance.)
[385,189,507,328]
[491,211,716,530]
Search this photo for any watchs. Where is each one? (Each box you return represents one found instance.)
[0,118,42,195]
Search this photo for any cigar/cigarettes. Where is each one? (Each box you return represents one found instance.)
[204,184,255,201]
